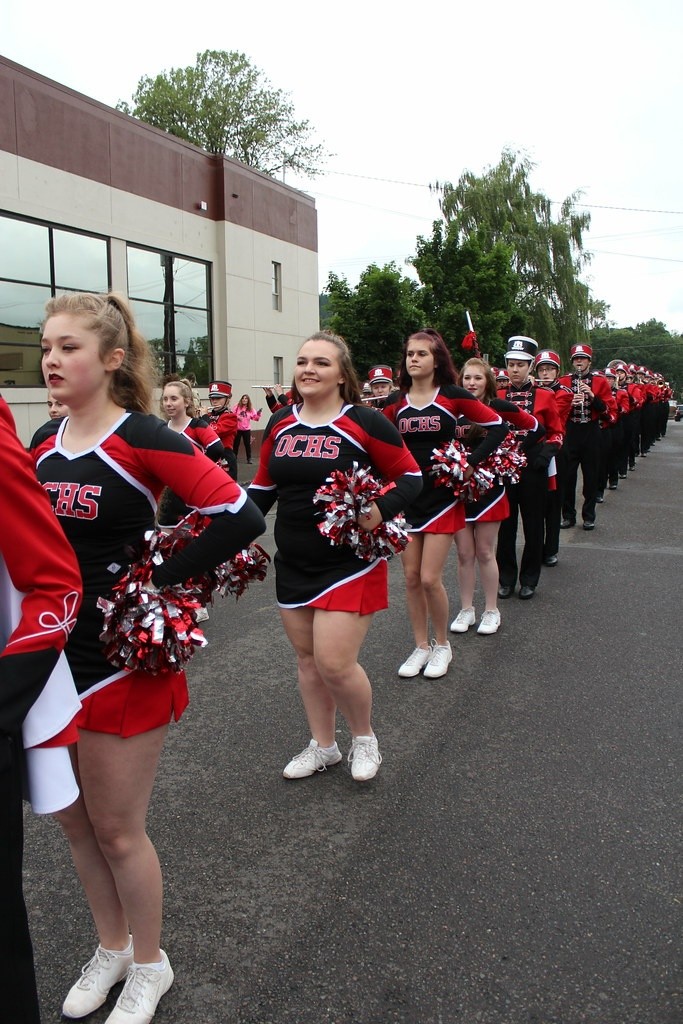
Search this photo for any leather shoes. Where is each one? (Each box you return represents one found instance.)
[582,521,595,530]
[635,434,666,458]
[542,553,559,566]
[560,517,576,529]
[596,465,636,503]
[500,585,537,600]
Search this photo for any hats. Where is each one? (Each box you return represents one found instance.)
[592,363,669,387]
[570,344,593,361]
[505,337,538,361]
[534,350,561,368]
[362,382,372,393]
[490,367,509,381]
[208,380,232,398]
[368,366,393,387]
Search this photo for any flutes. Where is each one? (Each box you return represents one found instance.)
[208,405,221,410]
[577,366,588,425]
[251,384,293,389]
[534,379,554,382]
[361,395,389,402]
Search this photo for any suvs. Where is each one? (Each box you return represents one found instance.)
[676,405,683,417]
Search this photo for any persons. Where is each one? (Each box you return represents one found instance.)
[246,330,424,781]
[0,393,83,1024]
[163,328,674,678]
[28,289,267,1024]
[45,389,70,420]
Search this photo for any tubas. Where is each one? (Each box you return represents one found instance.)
[657,380,665,387]
[664,384,668,390]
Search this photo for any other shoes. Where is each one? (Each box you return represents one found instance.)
[247,460,252,464]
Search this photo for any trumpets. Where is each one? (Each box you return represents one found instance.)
[614,376,620,387]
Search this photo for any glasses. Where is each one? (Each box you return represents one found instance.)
[537,367,558,372]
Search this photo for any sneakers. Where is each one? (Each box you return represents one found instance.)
[284,738,342,779]
[397,641,432,677]
[105,949,175,1024]
[62,934,134,1018]
[347,730,383,781]
[450,607,476,633]
[424,638,453,678]
[477,608,501,634]
